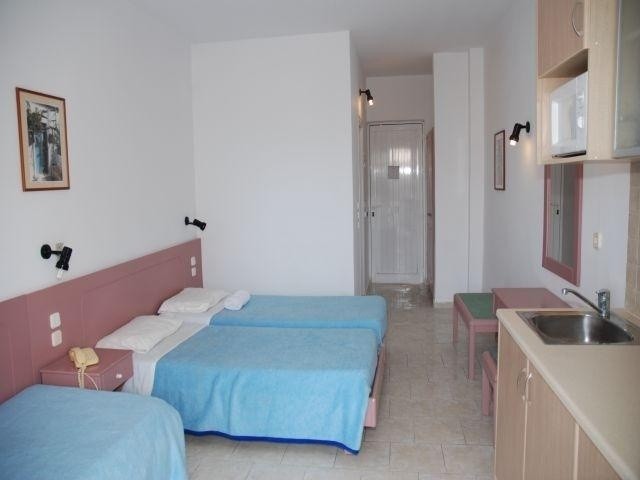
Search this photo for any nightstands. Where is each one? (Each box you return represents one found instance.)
[39,346,134,391]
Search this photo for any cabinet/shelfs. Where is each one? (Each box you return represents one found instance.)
[574,425,622,480]
[537,0,640,163]
[493,322,529,480]
[523,361,579,480]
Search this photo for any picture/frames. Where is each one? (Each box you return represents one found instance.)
[16,86,70,192]
[494,130,506,191]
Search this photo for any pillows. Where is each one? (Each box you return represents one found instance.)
[95,315,183,354]
[224,291,250,310]
[158,288,225,313]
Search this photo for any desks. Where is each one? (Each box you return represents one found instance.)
[452,292,499,383]
[492,288,573,309]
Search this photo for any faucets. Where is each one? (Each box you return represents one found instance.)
[561,287,610,317]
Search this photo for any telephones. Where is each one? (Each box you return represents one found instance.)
[68,347,100,389]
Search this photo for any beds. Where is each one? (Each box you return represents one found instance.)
[160,286,389,342]
[98,315,378,456]
[0,382,189,479]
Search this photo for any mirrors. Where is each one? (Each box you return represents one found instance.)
[541,164,583,288]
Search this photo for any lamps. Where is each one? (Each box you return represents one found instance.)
[509,122,530,147]
[359,88,374,106]
[186,216,208,234]
[41,245,73,280]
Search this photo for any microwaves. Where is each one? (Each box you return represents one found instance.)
[549,71,588,157]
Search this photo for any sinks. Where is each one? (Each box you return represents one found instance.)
[516,309,636,345]
